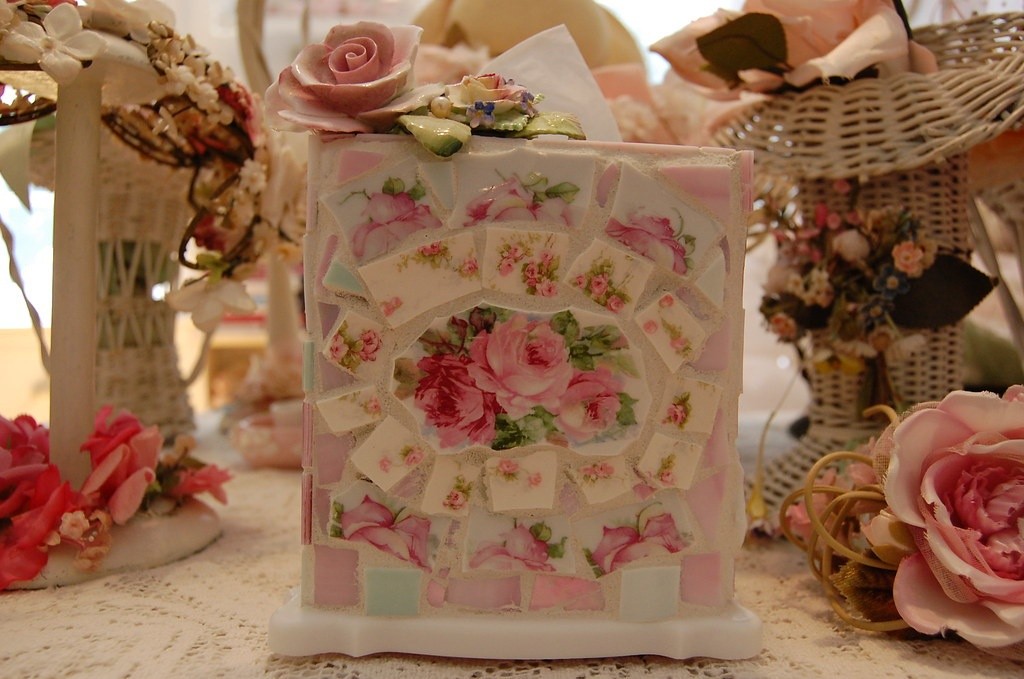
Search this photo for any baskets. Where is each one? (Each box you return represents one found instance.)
[20,104,216,449]
[710,12,1024,536]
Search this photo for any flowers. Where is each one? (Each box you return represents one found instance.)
[791,384,1024,665]
[0,404,233,594]
[1,0,295,330]
[747,173,1000,429]
[647,0,939,101]
[262,20,587,158]
